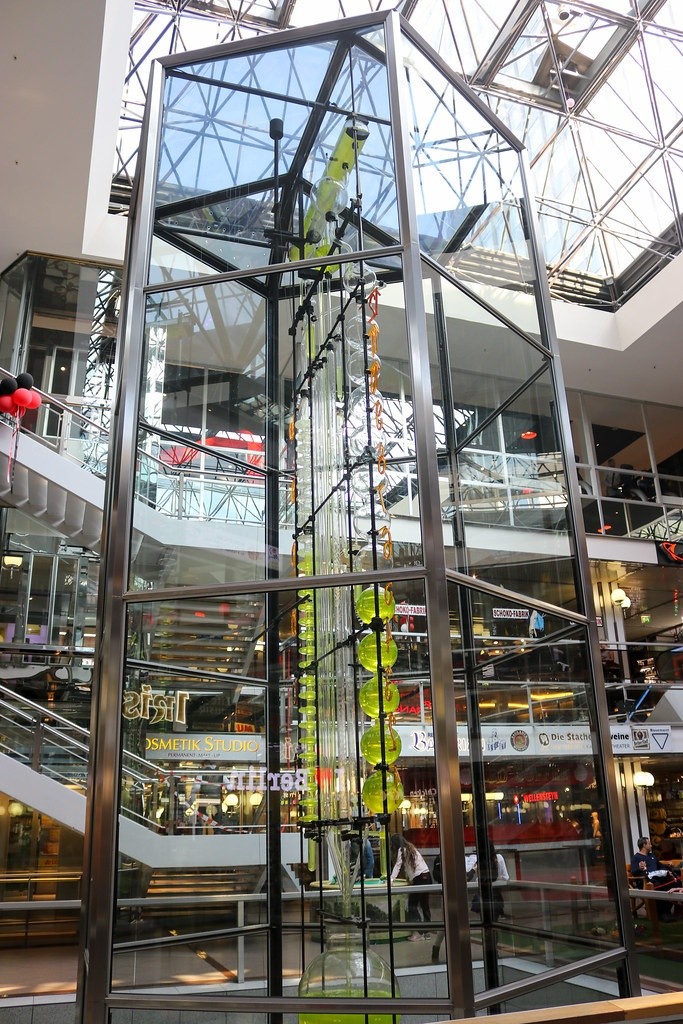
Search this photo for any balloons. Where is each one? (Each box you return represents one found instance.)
[0,372,42,419]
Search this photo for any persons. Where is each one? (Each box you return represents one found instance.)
[192,759,231,835]
[470,838,509,948]
[630,837,682,922]
[432,848,477,960]
[389,834,432,941]
[330,835,373,884]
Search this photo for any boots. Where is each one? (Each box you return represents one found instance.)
[432,945,440,964]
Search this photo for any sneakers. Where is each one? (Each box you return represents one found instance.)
[407,930,431,942]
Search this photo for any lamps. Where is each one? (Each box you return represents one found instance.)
[250,793,262,813]
[226,794,238,816]
[611,588,631,619]
[633,771,654,789]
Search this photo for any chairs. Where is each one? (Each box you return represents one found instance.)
[625,859,683,919]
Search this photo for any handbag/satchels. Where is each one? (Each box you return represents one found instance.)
[432,854,443,884]
